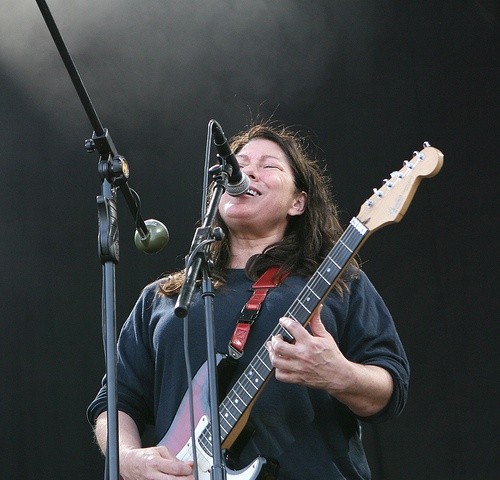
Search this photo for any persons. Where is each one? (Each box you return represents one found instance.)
[87,121,411,480]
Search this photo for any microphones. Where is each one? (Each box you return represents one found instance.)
[134,219,169,255]
[212,120,250,197]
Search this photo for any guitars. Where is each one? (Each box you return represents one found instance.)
[157,141,444,480]
